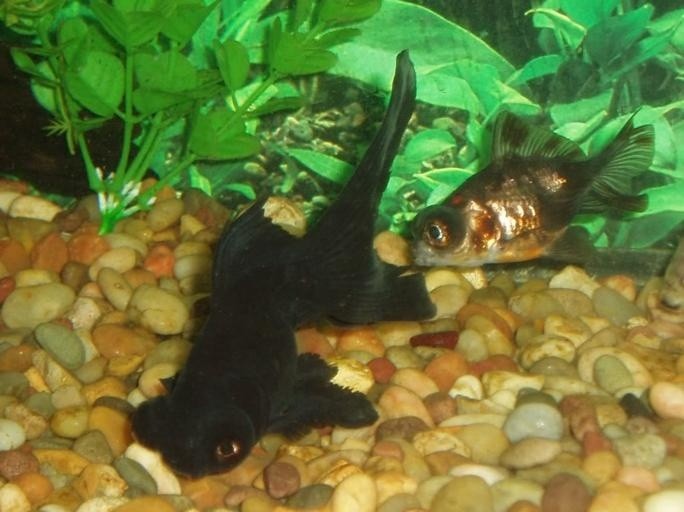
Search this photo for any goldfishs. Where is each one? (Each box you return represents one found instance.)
[408,107,677,268]
[127,187,380,480]
[210,47,438,330]
